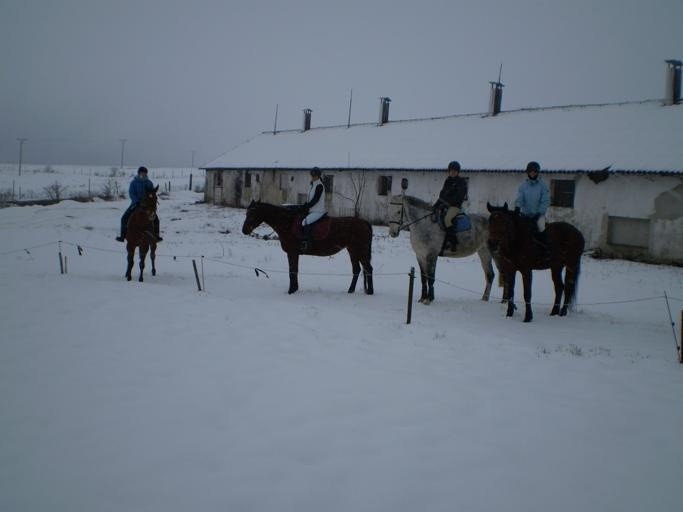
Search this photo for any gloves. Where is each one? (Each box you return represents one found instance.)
[514,207,521,217]
[532,212,540,222]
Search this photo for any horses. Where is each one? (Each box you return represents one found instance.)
[485,200,585,323]
[240,197,374,296]
[386,189,495,305]
[121,183,161,282]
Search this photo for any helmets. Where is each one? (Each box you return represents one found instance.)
[526,162,540,173]
[138,167,147,174]
[311,167,321,176]
[448,161,460,171]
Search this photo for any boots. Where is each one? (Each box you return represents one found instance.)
[447,225,457,252]
[301,229,313,254]
[537,229,553,262]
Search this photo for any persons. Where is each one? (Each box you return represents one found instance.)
[296,167,327,255]
[515,163,551,259]
[439,161,467,248]
[116,167,162,242]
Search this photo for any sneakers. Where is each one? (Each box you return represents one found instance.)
[116,236,124,242]
[156,237,163,242]
